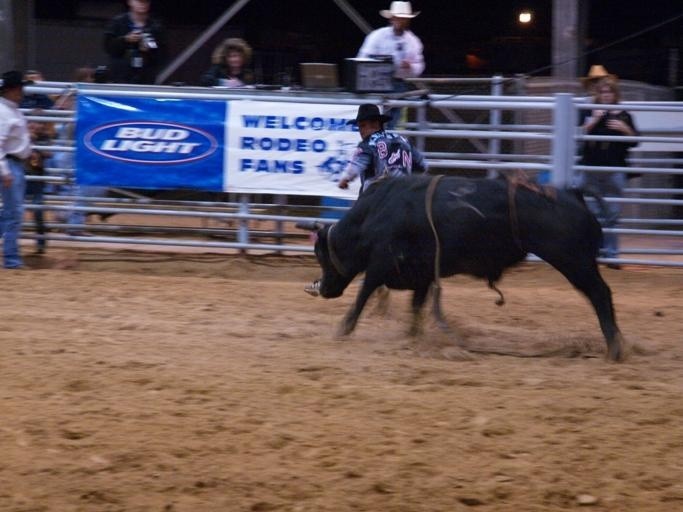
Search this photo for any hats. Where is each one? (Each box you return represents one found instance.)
[380,0,421,18]
[353,103,394,125]
[0,70,33,88]
[212,38,253,65]
[581,64,617,81]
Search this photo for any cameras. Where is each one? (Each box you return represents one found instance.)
[139,29,157,50]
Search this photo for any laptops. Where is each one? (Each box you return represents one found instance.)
[299,62,347,92]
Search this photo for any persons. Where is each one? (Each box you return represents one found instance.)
[103,0,158,85]
[202,38,257,88]
[578,25,614,76]
[575,64,640,270]
[502,25,552,77]
[337,104,430,285]
[0,65,108,270]
[355,1,426,145]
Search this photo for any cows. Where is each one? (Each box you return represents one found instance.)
[294,173,626,362]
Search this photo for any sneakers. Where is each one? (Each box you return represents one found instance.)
[304,279,321,295]
